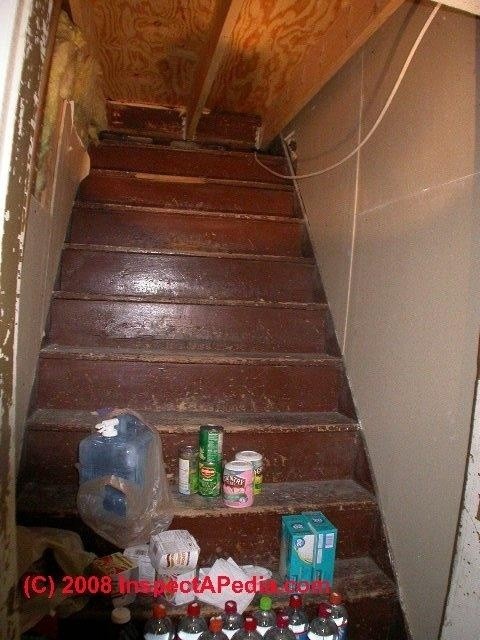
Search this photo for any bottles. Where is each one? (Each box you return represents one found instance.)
[178,424,264,509]
[109,593,348,640]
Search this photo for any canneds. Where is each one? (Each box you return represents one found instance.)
[197,426,224,498]
[222,460,254,508]
[178,445,200,496]
[235,450,264,496]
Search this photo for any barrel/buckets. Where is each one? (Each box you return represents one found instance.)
[78,414,152,526]
